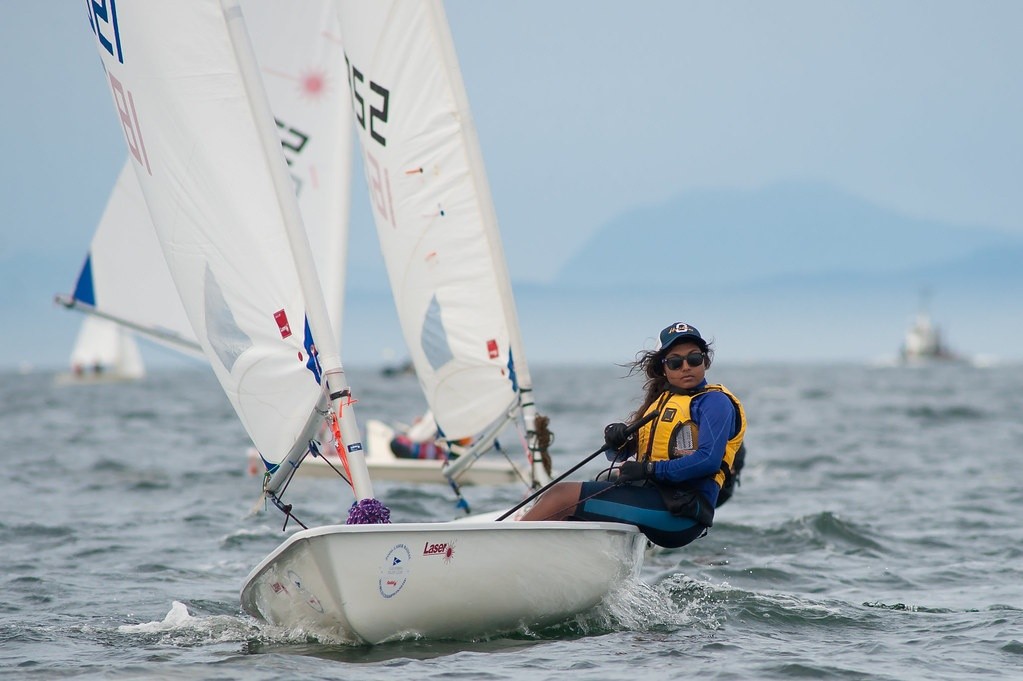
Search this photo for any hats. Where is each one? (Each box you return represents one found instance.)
[659,321,706,351]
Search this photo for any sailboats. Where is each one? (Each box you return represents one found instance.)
[46,0,646,643]
[54,314,146,386]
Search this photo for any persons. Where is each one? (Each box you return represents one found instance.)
[519,322,747,547]
[390,417,445,460]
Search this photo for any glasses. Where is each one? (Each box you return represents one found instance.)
[660,352,710,371]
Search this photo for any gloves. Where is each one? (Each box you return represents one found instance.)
[604,423,628,448]
[614,461,657,486]
